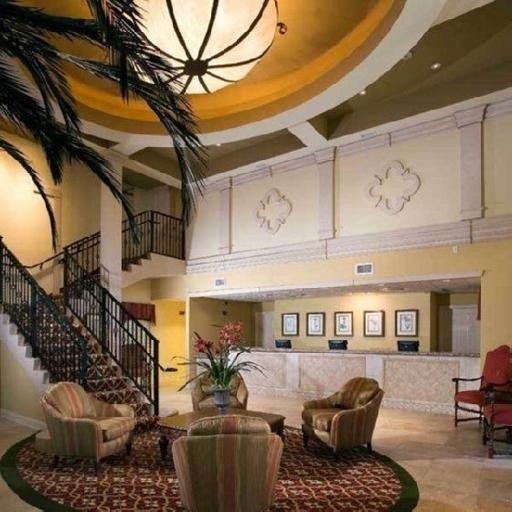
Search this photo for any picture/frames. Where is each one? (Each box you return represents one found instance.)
[363,310,386,337]
[334,311,353,336]
[306,312,325,336]
[394,309,419,337]
[281,313,300,336]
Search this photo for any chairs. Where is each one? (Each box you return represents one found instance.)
[481,389,512,459]
[452,345,512,426]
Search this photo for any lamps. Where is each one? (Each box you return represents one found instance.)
[124,0,287,94]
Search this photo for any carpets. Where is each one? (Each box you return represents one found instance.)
[0,413,418,511]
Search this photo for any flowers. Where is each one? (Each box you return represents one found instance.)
[171,320,267,391]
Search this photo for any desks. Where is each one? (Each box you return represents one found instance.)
[156,406,287,461]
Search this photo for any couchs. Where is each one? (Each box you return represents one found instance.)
[172,415,284,512]
[301,377,384,465]
[40,381,137,476]
[191,370,249,411]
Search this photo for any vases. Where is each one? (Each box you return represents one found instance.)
[212,389,231,416]
